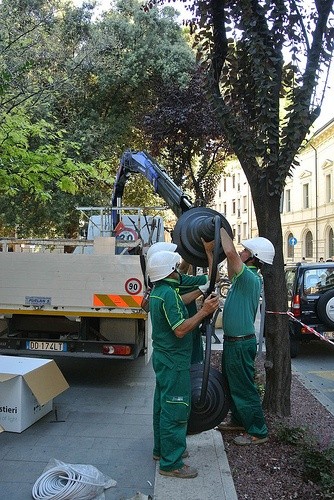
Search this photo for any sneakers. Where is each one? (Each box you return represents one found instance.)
[160,464,197,477]
[233,433,268,445]
[218,422,245,430]
[153,450,189,460]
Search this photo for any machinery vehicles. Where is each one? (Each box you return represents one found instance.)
[0,148,200,366]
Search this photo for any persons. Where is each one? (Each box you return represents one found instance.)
[319,257,326,275]
[147,237,214,365]
[148,251,220,479]
[218,222,275,446]
[301,257,307,263]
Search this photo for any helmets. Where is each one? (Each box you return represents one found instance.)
[148,251,181,282]
[146,242,178,261]
[241,237,275,265]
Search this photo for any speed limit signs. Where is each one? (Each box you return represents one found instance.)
[125,278,142,295]
[115,228,138,250]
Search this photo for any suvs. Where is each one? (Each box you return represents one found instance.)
[284,260,334,358]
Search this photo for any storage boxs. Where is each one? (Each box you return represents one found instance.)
[0,356,70,433]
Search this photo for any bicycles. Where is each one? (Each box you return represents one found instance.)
[216,266,233,298]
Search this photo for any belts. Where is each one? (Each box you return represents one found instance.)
[223,334,255,341]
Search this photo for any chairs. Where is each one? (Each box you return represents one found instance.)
[307,275,320,286]
